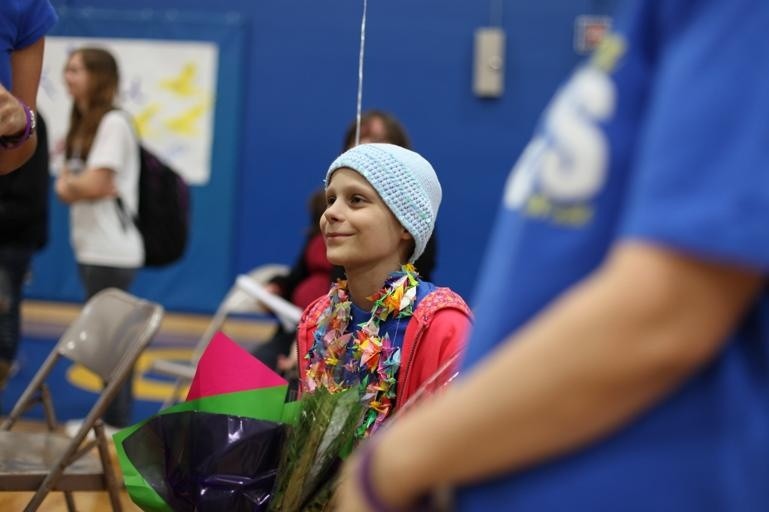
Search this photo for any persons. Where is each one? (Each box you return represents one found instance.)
[249,111,436,378]
[0,0,59,177]
[296,142,472,445]
[329,0,769,512]
[1,111,49,391]
[56,50,147,440]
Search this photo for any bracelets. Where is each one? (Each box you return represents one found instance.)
[356,440,400,512]
[0,97,38,149]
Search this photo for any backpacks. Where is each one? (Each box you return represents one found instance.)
[113,145,190,269]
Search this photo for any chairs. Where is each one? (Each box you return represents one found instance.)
[0,288,164,507]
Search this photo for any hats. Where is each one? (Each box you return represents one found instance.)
[324,141,443,263]
